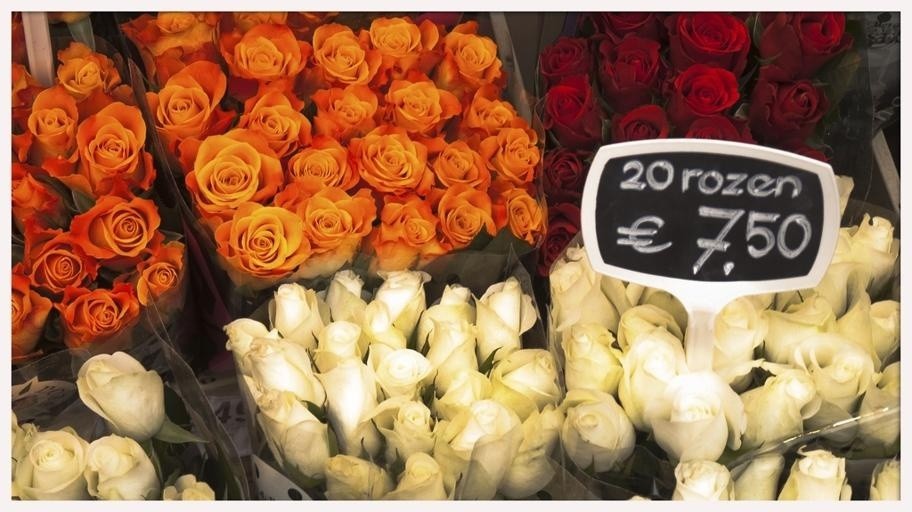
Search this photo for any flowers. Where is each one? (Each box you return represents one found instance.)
[13,12,899,499]
